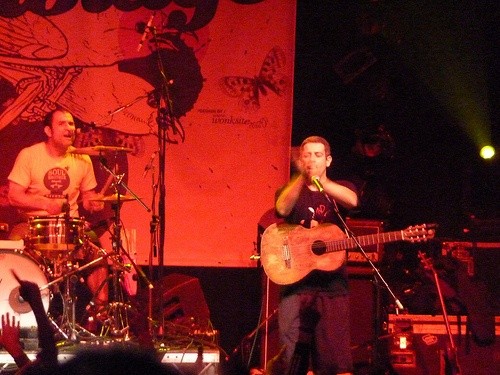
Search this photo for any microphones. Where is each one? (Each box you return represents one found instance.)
[311,175,324,193]
[84,215,115,232]
[77,122,96,133]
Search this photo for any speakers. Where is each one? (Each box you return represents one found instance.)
[438,242,500,313]
[262,265,382,374]
[386,313,500,375]
[134,272,210,336]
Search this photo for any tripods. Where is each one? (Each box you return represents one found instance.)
[83,161,154,342]
[46,258,97,341]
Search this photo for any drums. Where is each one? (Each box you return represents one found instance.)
[0,227,26,251]
[0,245,55,330]
[26,214,86,250]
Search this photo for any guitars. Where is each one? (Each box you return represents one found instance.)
[260,222,436,286]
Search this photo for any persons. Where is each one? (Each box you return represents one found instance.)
[7,111,109,334]
[275,136,359,373]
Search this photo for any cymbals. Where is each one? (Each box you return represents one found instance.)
[65,146,134,157]
[87,193,144,206]
